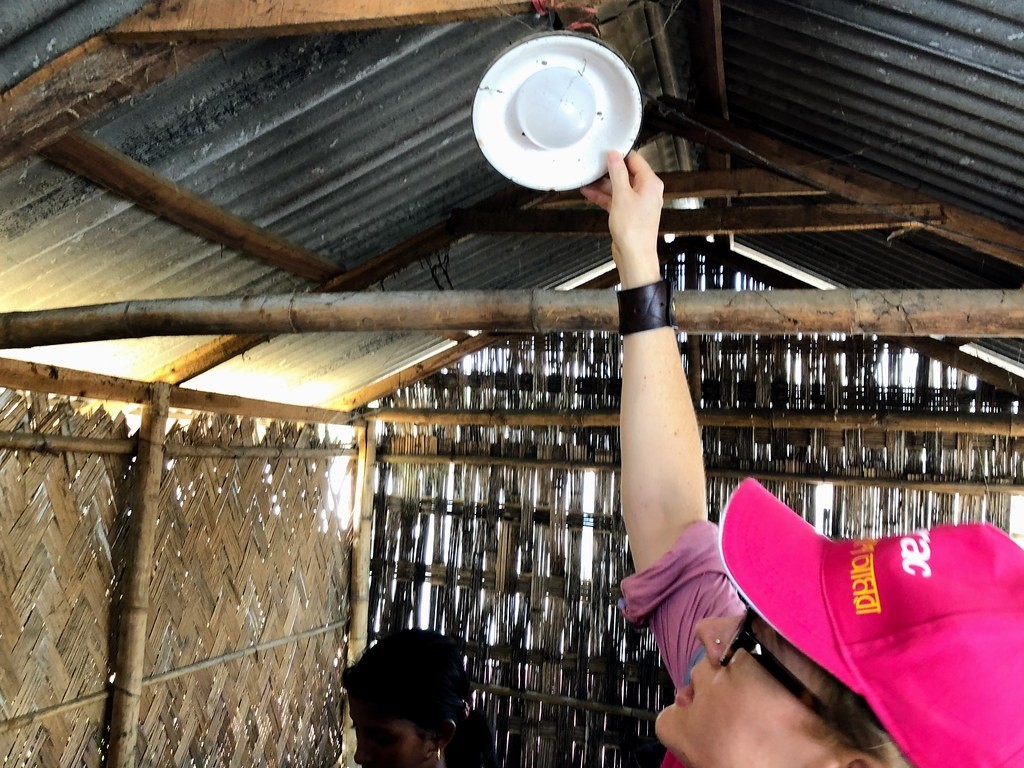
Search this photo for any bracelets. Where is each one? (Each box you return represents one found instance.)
[616,277,679,335]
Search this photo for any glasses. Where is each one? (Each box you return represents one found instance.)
[720,608,857,741]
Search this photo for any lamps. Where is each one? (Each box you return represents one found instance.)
[471,11,643,193]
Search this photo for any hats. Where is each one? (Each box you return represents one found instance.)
[717,478,1024,768]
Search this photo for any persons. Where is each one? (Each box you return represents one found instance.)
[579,146,1024,768]
[340,628,498,768]
